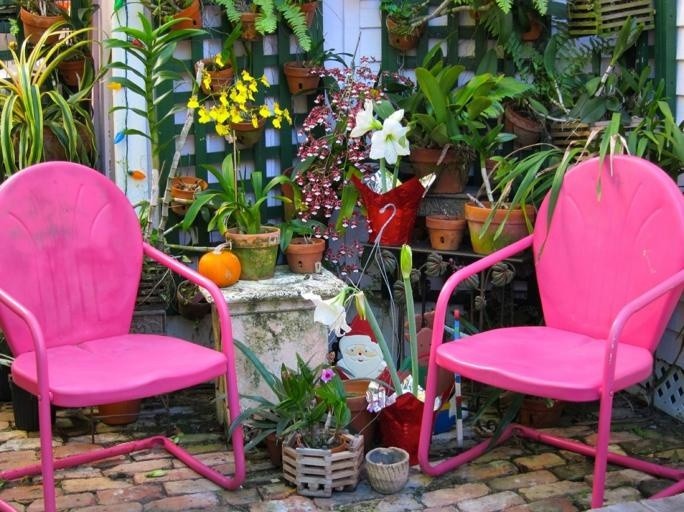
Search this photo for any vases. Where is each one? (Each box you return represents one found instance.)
[97,401,142,425]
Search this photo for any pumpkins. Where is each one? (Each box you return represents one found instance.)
[199,251,241,288]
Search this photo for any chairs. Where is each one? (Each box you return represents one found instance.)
[0,161,245,512]
[418,153,684,508]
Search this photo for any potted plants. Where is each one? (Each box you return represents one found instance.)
[1,0,679,321]
[210,202,568,497]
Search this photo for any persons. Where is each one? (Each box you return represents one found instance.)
[328,311,410,397]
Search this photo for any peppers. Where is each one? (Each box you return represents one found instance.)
[128,170,145,180]
[106,83,125,89]
[128,39,145,48]
